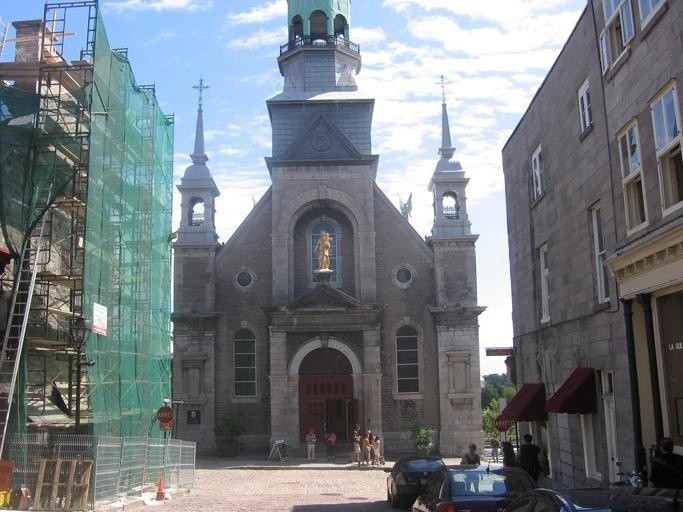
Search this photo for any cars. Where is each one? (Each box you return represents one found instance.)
[382,454,448,507]
[497,486,683,512]
[410,459,534,511]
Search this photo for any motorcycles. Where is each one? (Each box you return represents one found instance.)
[607,456,649,487]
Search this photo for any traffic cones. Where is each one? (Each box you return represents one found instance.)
[154,476,165,501]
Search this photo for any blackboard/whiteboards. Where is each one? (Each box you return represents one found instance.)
[276,441,289,461]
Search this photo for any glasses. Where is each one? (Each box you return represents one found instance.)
[469,447,474,449]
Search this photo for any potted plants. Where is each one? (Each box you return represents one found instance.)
[214,407,246,456]
[413,424,440,457]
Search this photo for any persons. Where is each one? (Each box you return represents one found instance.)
[644,436,683,494]
[325,429,338,463]
[412,465,457,511]
[313,231,334,269]
[491,434,500,463]
[461,443,483,492]
[515,435,545,486]
[304,427,317,462]
[353,422,381,468]
[503,442,516,467]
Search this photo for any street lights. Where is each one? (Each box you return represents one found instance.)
[68,315,91,433]
[162,396,170,477]
